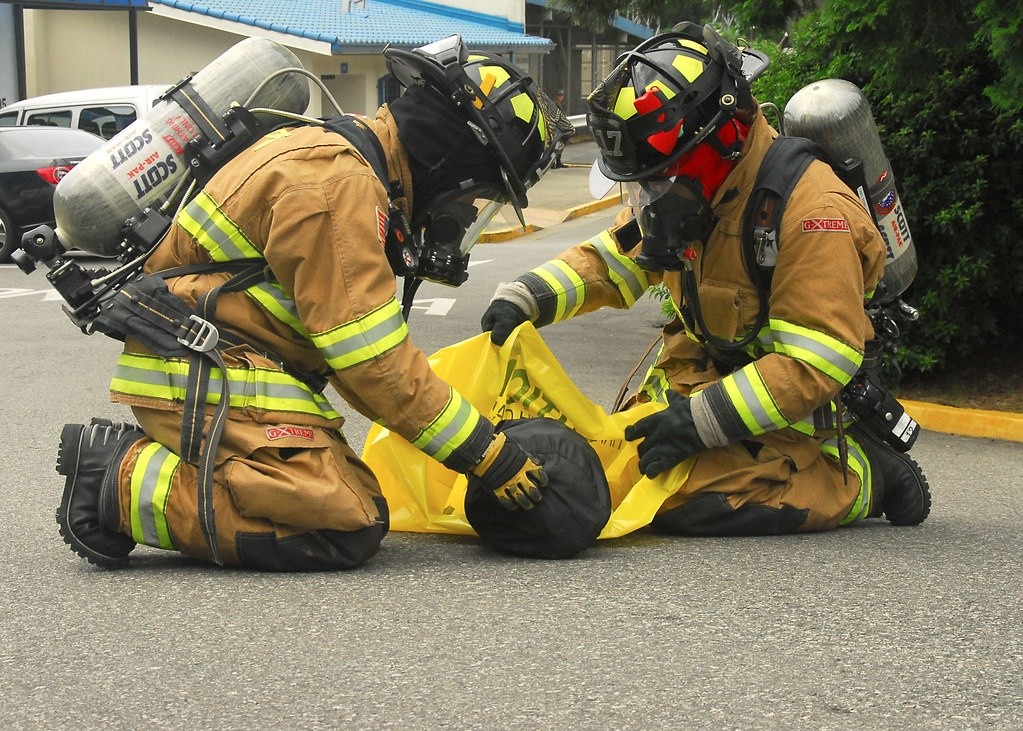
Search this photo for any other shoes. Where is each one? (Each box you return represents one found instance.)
[556,162,568,168]
[551,164,556,169]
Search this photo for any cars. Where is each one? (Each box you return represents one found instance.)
[0,122,109,266]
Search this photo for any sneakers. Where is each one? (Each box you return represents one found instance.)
[842,417,931,526]
[55,417,146,569]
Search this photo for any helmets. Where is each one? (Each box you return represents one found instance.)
[554,88,565,95]
[587,22,770,184]
[383,32,574,208]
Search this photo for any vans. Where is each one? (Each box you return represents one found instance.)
[0,83,176,144]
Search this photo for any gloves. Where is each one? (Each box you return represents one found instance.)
[624,389,728,479]
[481,280,541,346]
[465,432,548,511]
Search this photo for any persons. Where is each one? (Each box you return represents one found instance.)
[550,89,568,169]
[480,21,933,536]
[55,33,576,573]
